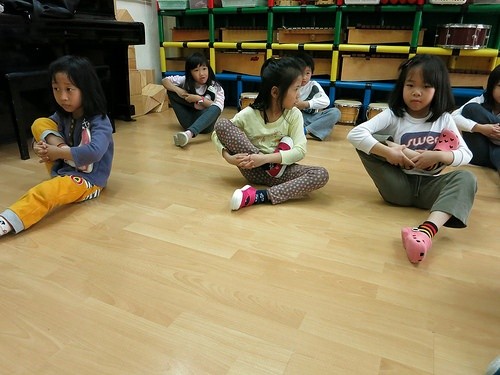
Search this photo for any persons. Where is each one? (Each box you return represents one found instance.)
[161,52,226,149]
[346,54,479,265]
[290,55,342,141]
[212,55,331,212]
[450,64,500,174]
[1,55,116,238]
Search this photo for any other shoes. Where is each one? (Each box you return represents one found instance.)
[229,184,257,211]
[266,137,294,179]
[74,128,93,173]
[203,85,217,104]
[172,131,190,147]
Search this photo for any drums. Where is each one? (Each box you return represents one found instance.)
[434,21,493,50]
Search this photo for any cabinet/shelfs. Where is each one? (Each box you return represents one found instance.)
[156,1,499,127]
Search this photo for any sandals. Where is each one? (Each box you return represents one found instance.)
[434,127,459,152]
[401,226,432,265]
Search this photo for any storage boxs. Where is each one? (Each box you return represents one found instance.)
[342,56,407,81]
[275,27,340,44]
[446,71,489,90]
[114,8,168,117]
[344,26,427,47]
[216,52,266,76]
[367,103,389,121]
[220,27,273,44]
[312,59,331,77]
[171,26,219,42]
[158,0,499,10]
[449,57,497,74]
[334,100,361,126]
[240,92,258,110]
